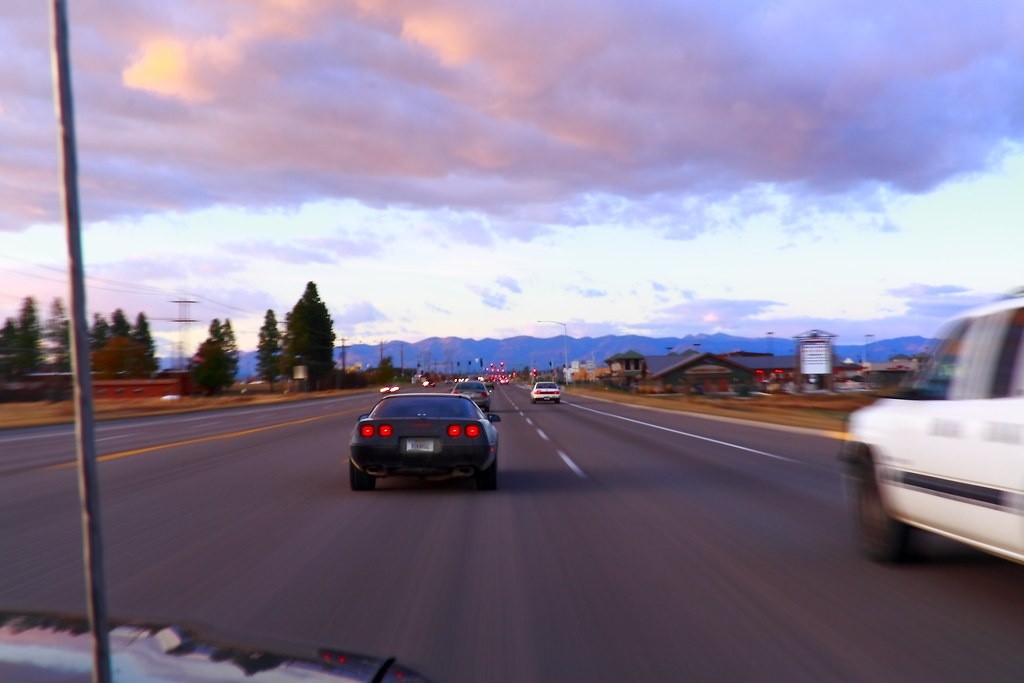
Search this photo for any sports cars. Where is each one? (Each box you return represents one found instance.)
[347,392,502,492]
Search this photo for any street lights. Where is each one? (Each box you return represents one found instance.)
[537,320,568,386]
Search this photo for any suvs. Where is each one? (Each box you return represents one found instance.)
[836,281,1023,572]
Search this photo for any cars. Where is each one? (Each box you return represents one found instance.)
[529,381,561,404]
[449,380,492,413]
